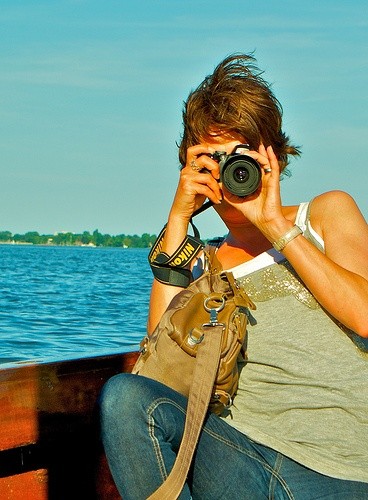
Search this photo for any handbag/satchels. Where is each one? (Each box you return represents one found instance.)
[131,246,255,418]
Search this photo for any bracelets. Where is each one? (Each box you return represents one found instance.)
[273,225,303,253]
[191,160,201,173]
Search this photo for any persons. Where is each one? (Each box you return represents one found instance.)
[98,52,368,500]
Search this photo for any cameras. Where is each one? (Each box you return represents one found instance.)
[197,143,261,197]
